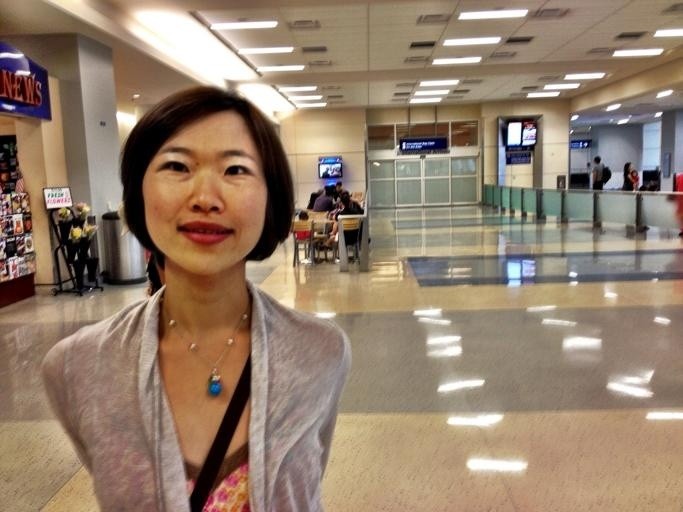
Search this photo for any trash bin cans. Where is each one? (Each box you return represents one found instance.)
[101,212,148,284]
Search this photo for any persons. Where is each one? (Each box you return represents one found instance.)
[669,172,683,237]
[591,156,610,190]
[41,88,352,510]
[296,177,362,265]
[630,170,638,190]
[622,163,634,190]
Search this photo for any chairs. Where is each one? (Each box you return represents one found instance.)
[292,191,365,267]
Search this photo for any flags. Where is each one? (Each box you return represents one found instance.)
[14,172,23,194]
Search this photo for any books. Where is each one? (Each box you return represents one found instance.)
[0,192,38,281]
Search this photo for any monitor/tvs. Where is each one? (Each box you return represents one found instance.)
[505,120,537,147]
[318,163,342,178]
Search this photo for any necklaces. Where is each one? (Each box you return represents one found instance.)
[161,299,251,396]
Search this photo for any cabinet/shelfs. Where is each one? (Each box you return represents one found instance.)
[0,191,37,309]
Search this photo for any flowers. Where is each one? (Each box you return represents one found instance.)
[57,202,96,244]
